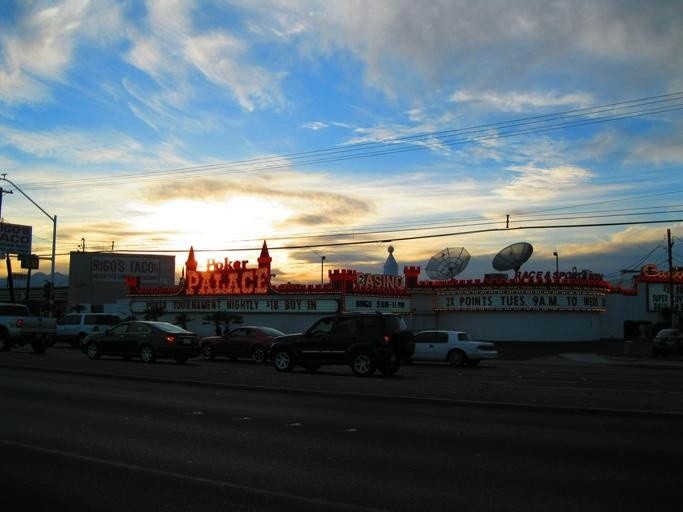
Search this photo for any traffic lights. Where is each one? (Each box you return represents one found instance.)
[43,283,51,298]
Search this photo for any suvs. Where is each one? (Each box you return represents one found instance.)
[266,310,416,377]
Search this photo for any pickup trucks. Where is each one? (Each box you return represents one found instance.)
[413,329,499,367]
[0,303,57,353]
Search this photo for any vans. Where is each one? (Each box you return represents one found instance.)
[49,313,120,349]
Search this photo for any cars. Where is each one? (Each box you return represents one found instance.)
[80,321,202,365]
[652,328,682,357]
[199,326,288,363]
